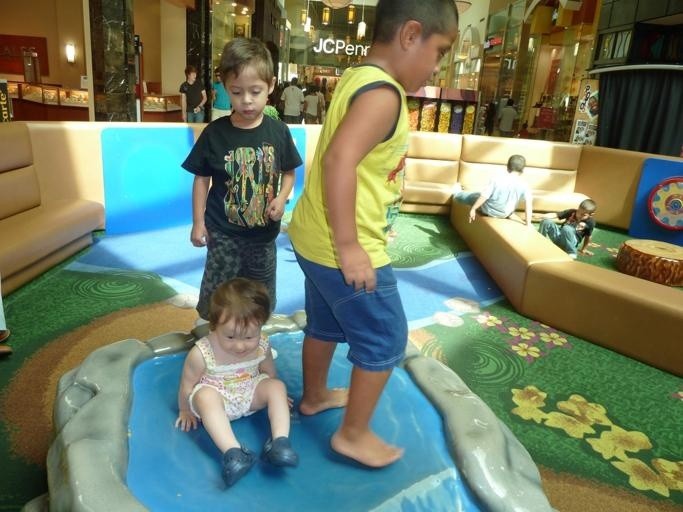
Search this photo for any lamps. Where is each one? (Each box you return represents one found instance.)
[65,43,75,64]
[301,0,366,41]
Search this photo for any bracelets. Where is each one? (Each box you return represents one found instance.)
[198,105,203,110]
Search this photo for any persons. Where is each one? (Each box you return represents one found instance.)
[483,103,496,136]
[267,76,333,124]
[181,38,303,359]
[532,199,596,259]
[498,99,519,137]
[518,123,529,138]
[0,294,13,354]
[209,66,233,122]
[454,155,534,229]
[289,0,459,468]
[179,65,208,123]
[174,278,298,487]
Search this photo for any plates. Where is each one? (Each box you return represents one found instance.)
[582,90,599,120]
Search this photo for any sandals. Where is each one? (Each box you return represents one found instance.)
[220,443,258,487]
[259,437,299,467]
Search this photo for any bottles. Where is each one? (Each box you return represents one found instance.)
[211,86,216,102]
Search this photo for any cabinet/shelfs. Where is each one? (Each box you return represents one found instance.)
[140,94,185,122]
[6,81,89,121]
